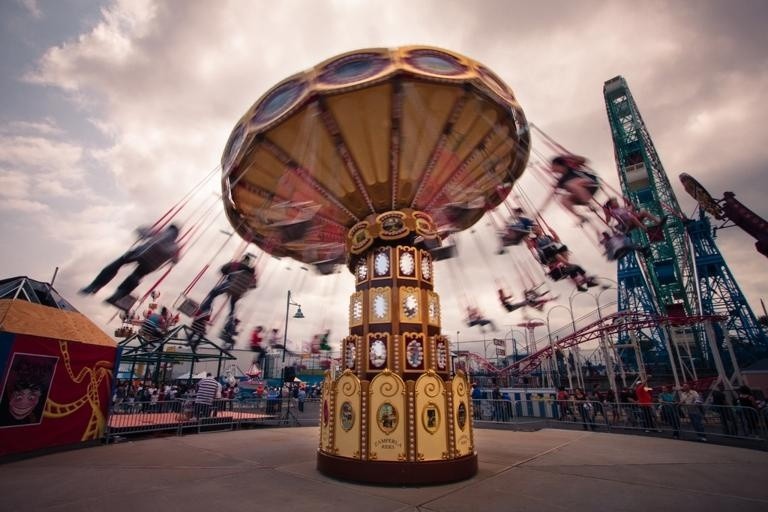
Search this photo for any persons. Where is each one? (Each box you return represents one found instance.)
[78,154,767,441]
